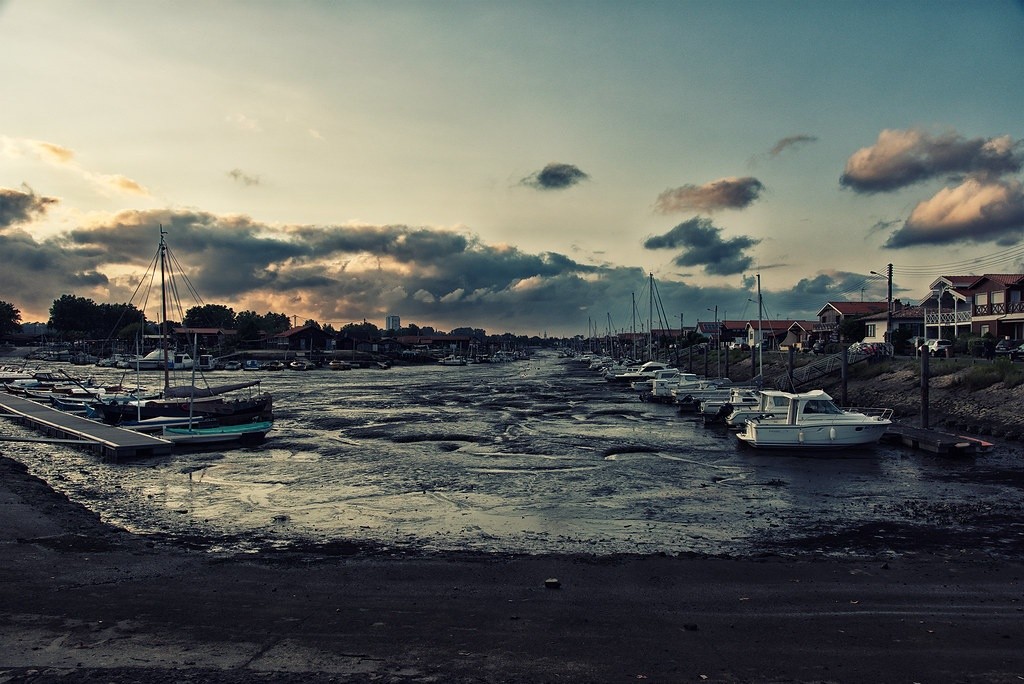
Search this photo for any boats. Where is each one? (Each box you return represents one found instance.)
[736,389,894,449]
[162,333,273,447]
[0,361,159,412]
[631,368,680,390]
[644,374,724,399]
[670,378,758,401]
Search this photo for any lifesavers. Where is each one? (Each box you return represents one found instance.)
[182,404,189,410]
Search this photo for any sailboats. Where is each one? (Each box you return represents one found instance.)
[32,313,393,371]
[554,270,811,422]
[58,222,273,428]
[402,337,535,364]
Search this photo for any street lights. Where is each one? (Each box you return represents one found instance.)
[748,299,763,389]
[673,315,683,348]
[729,344,744,351]
[870,270,893,355]
[707,308,717,348]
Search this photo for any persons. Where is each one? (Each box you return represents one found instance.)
[813,341,820,355]
[865,344,876,355]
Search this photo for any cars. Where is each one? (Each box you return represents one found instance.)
[915,338,954,357]
[995,340,1024,361]
[754,342,767,350]
[848,342,877,356]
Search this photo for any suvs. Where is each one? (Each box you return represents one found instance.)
[813,341,827,354]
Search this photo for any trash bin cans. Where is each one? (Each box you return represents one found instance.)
[945,345,955,358]
